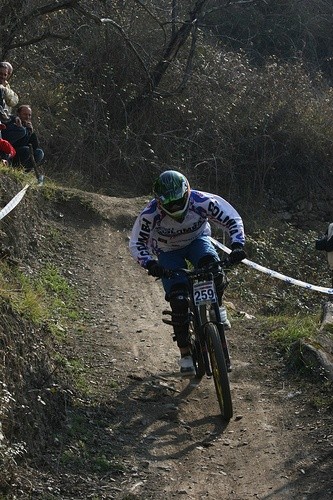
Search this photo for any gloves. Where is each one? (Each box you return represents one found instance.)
[145,260,168,279]
[229,242,247,263]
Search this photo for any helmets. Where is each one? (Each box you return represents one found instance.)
[153,171,191,220]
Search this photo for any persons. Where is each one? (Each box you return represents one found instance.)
[3,104,45,173]
[0,120,16,167]
[126,169,248,376]
[0,61,19,127]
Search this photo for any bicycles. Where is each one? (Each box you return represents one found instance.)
[149,256,239,420]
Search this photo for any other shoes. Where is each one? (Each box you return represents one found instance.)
[209,307,231,330]
[179,346,196,379]
